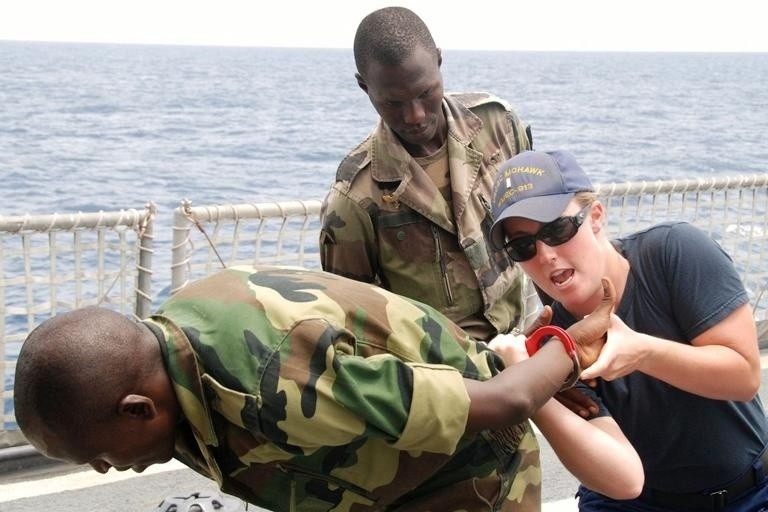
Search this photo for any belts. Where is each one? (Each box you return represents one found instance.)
[640,447,768,511]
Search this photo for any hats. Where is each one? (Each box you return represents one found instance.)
[489,149,595,252]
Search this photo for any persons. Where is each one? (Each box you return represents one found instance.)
[485,149,764,510]
[15,271,617,510]
[316,7,534,353]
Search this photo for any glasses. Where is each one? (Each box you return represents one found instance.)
[501,204,593,262]
